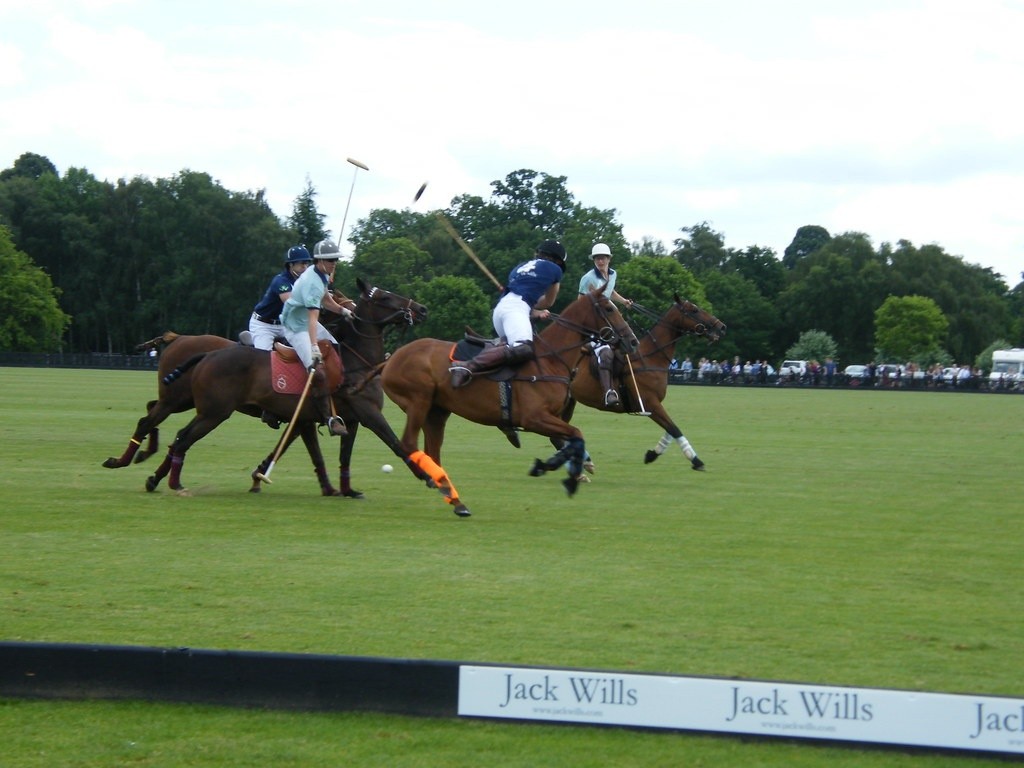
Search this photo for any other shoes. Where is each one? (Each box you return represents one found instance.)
[262,411,280,429]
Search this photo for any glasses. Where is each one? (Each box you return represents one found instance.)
[322,258,338,262]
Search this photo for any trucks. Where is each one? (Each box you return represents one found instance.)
[989,348,1024,386]
[875,365,925,379]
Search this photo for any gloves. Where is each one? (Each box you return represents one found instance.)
[343,309,354,319]
[312,345,323,361]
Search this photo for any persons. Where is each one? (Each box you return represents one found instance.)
[577,243,634,407]
[249,246,315,430]
[279,239,356,436]
[149,348,158,357]
[672,356,1024,391]
[451,239,567,389]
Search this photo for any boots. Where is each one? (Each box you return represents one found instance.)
[450,345,508,391]
[308,362,348,435]
[598,347,619,410]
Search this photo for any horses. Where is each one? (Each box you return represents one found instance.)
[498,290,728,472]
[348,281,639,498]
[101,277,472,517]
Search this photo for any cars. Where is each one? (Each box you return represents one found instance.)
[843,365,871,380]
[934,368,968,378]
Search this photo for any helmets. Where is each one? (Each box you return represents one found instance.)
[536,238,567,273]
[312,240,344,259]
[588,243,613,260]
[285,246,313,261]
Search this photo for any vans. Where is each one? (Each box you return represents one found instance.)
[780,360,808,377]
